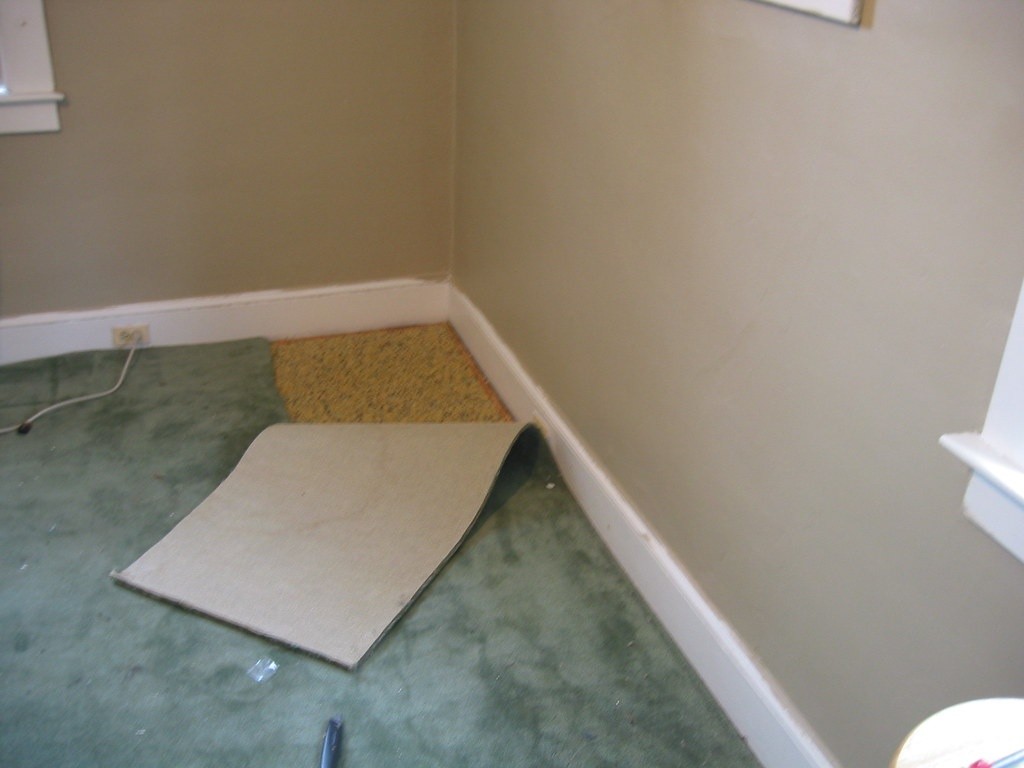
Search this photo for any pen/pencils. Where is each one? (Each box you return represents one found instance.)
[321,717,343,768]
